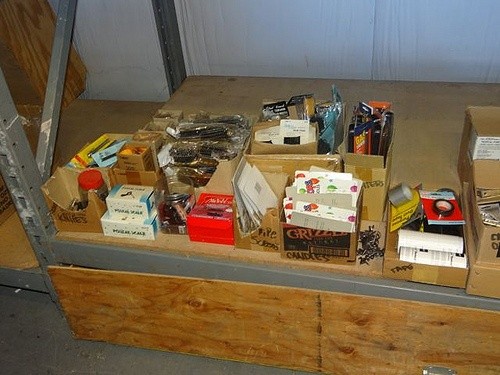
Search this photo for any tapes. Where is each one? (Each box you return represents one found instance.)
[432,197,454,218]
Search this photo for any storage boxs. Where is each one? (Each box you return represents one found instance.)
[468,159,500,264]
[40,109,393,266]
[460,183,500,299]
